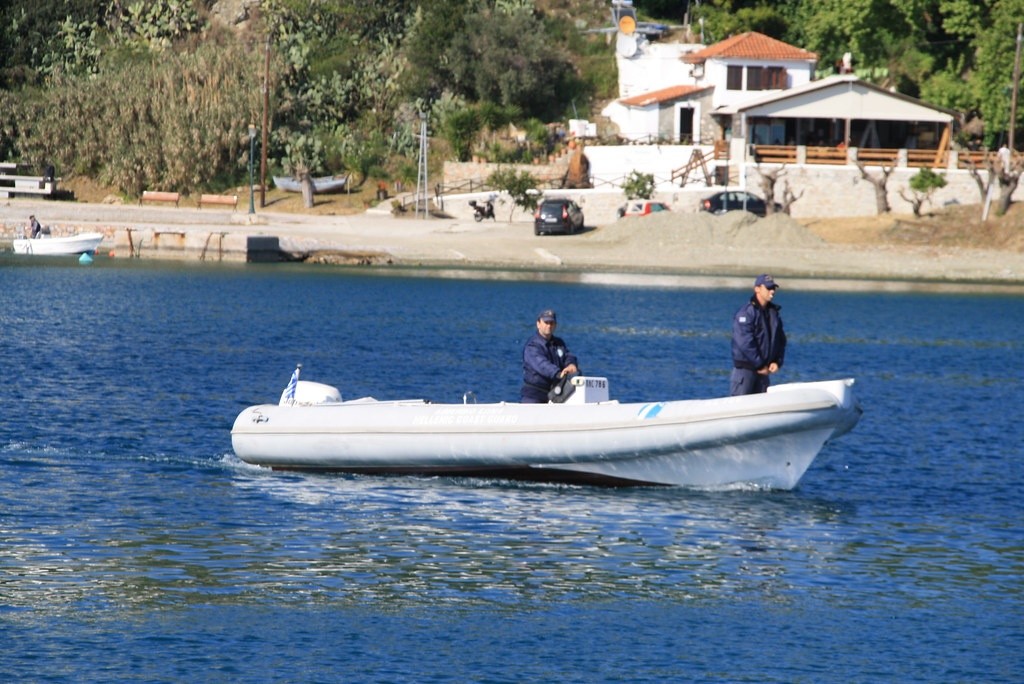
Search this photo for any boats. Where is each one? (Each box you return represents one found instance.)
[272,173,349,194]
[11,232,106,257]
[229,376,862,492]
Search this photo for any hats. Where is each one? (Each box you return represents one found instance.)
[539,309,555,321]
[755,275,779,289]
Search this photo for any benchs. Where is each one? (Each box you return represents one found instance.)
[139,191,179,208]
[196,194,238,212]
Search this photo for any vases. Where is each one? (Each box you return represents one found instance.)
[548,141,576,160]
[533,158,540,165]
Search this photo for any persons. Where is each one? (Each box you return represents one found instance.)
[518,309,582,402]
[25,215,41,239]
[730,274,787,395]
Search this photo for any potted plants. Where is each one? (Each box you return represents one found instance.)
[363,198,373,208]
[479,152,489,163]
[471,152,480,163]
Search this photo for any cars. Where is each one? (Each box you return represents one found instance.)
[701,190,784,218]
[532,199,585,236]
[616,199,670,220]
